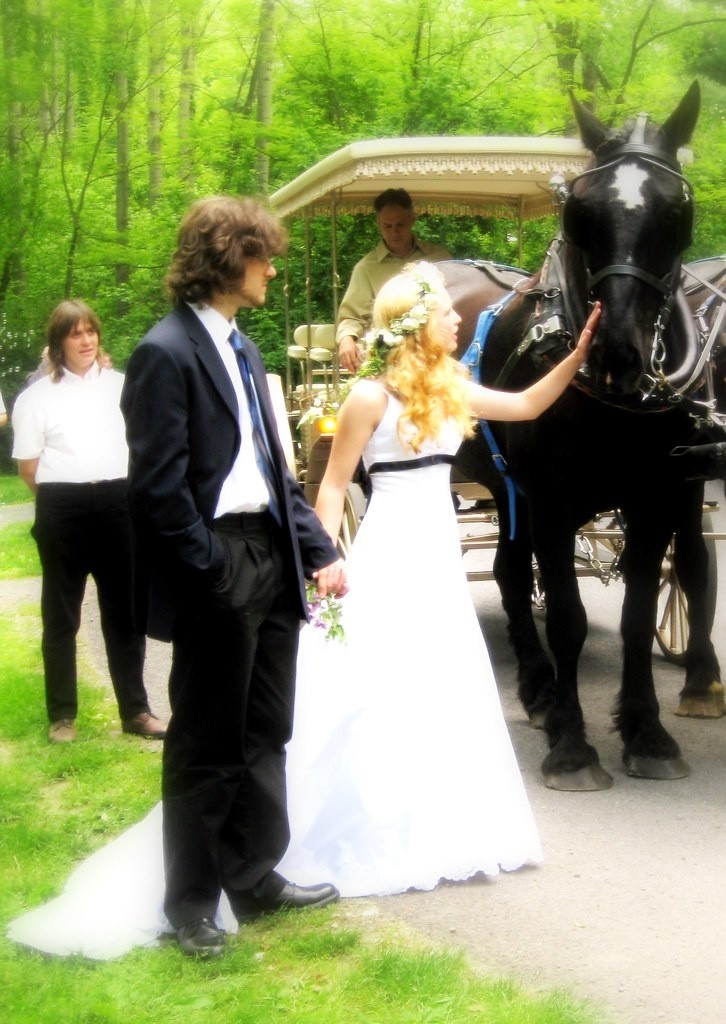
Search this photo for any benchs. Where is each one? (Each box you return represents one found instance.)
[285,324,355,400]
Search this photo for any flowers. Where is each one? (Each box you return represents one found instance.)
[301,575,348,643]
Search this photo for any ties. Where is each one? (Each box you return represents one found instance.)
[230,331,283,528]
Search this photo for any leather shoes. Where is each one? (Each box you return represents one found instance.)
[47,718,76,744]
[230,880,341,927]
[172,914,229,962]
[120,711,170,740]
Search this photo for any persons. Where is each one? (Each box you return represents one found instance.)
[12,300,180,743]
[120,194,338,960]
[337,189,465,368]
[277,261,602,898]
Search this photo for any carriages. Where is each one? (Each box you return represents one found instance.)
[252,78,726,795]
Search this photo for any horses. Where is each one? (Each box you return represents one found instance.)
[434,78,704,794]
[676,257,726,721]
